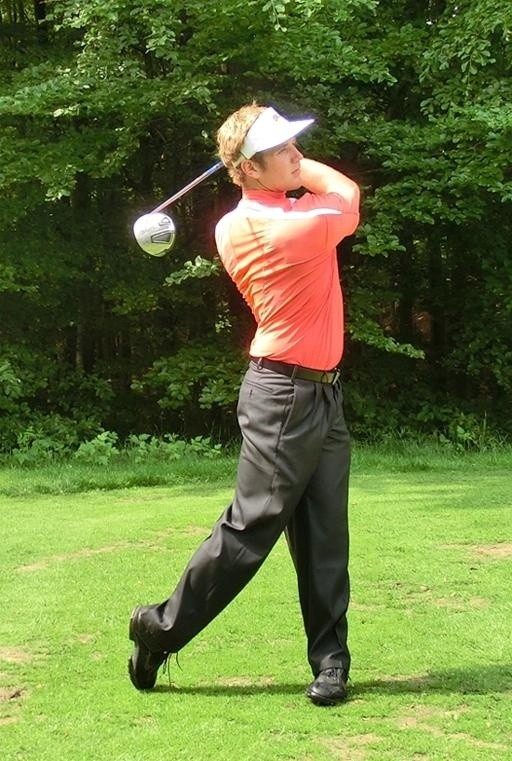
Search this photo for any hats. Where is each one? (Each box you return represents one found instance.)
[228,105,314,168]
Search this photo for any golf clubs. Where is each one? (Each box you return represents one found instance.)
[133,161,225,258]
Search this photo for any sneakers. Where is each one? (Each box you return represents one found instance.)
[305,666,354,705]
[125,604,183,691]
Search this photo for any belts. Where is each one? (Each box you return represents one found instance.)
[249,353,344,387]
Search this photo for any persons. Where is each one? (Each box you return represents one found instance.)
[127,103,360,708]
